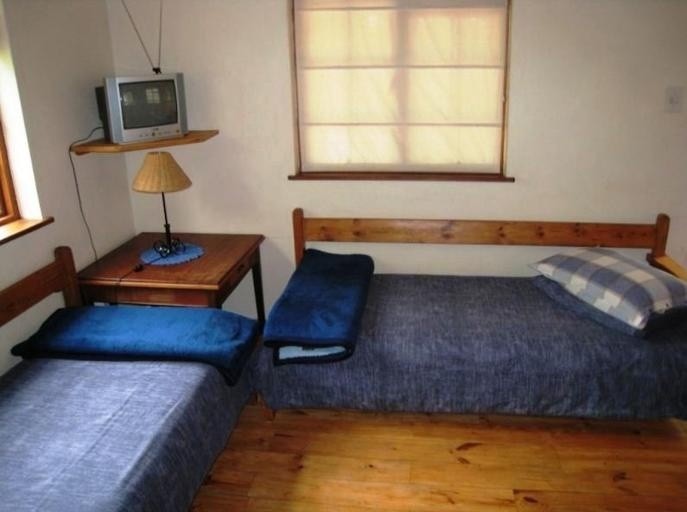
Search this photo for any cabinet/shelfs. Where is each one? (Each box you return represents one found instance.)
[70,130,221,155]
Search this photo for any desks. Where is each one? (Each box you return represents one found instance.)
[78,232,266,323]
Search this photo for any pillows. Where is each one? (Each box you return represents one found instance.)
[526,247,687,329]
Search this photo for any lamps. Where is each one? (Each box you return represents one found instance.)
[133,151,192,255]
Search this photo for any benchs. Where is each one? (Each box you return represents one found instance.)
[0,245,258,512]
[257,208,685,420]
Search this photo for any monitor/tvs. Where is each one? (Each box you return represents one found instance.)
[96,73,187,144]
